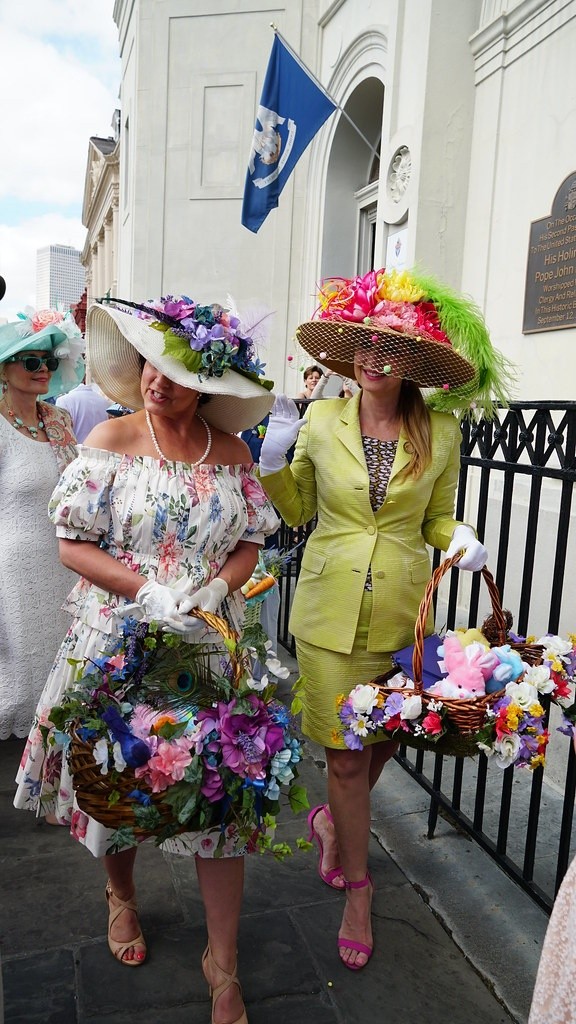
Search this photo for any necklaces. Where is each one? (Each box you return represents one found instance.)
[145,408,214,465]
[3,398,45,439]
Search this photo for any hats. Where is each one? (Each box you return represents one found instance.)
[86,303,274,435]
[1,322,85,400]
[297,320,477,390]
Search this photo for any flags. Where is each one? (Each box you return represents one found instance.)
[241,34,338,234]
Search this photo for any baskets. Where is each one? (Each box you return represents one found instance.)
[67,607,243,834]
[244,602,261,628]
[367,549,547,758]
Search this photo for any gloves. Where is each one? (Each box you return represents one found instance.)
[135,579,199,632]
[163,578,229,636]
[259,394,307,478]
[447,525,488,572]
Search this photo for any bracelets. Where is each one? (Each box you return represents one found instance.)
[323,373,329,379]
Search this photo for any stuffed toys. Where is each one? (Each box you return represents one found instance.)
[128,702,200,742]
[427,628,523,701]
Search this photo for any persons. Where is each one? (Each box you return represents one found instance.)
[1,306,90,829]
[8,293,283,1024]
[251,271,511,972]
[296,365,324,398]
[525,855,576,1024]
[45,378,113,445]
[309,369,363,398]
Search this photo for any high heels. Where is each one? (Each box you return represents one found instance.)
[104,878,146,966]
[202,942,248,1024]
[338,876,373,970]
[308,804,347,890]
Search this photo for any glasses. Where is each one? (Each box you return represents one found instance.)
[10,356,59,371]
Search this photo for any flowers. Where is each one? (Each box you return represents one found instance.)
[14,307,85,401]
[94,286,276,399]
[36,600,312,866]
[316,267,511,420]
[332,631,576,770]
